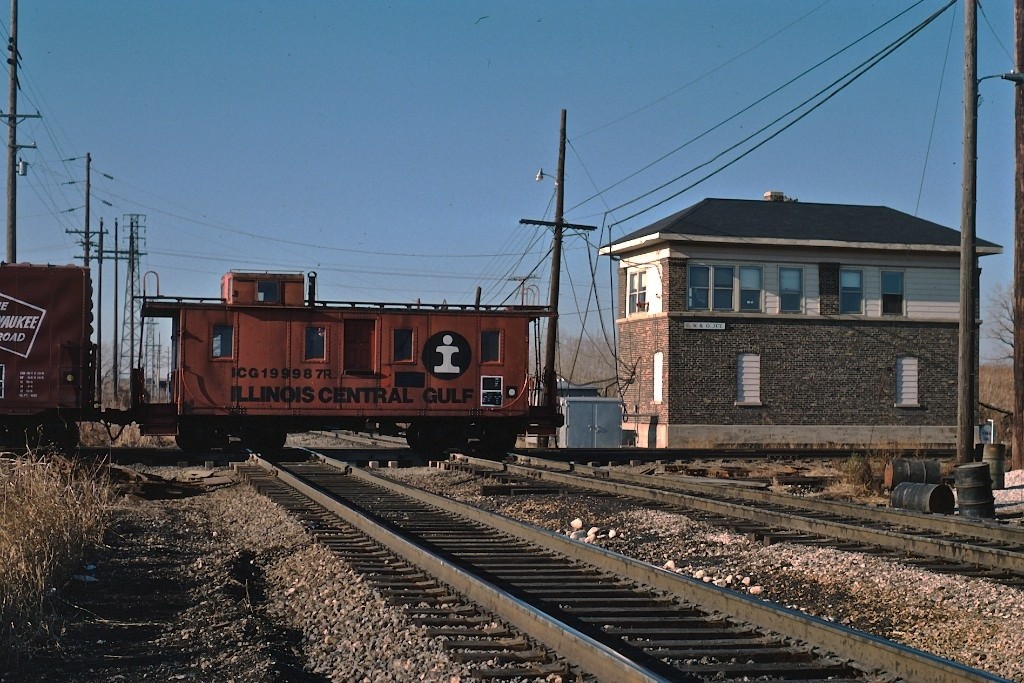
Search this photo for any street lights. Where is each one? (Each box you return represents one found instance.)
[533,169,571,450]
[955,71,1024,470]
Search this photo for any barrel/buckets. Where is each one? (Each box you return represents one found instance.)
[982,443,1005,490]
[884,458,941,490]
[954,462,995,520]
[892,482,955,515]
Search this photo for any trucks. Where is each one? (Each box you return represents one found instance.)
[0,262,565,457]
